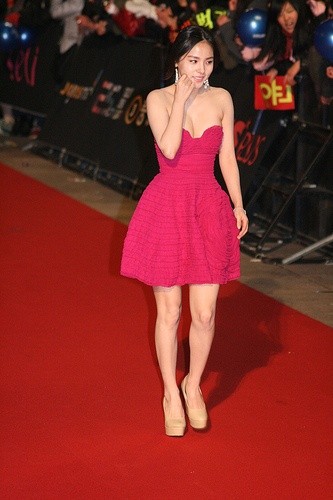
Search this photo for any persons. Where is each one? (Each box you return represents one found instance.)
[121,25,249,437]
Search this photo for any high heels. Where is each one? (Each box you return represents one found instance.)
[181,374,208,429]
[163,389,186,436]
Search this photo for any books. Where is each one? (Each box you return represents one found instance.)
[0,0,333,246]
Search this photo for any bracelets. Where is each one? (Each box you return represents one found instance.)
[233,208,246,215]
